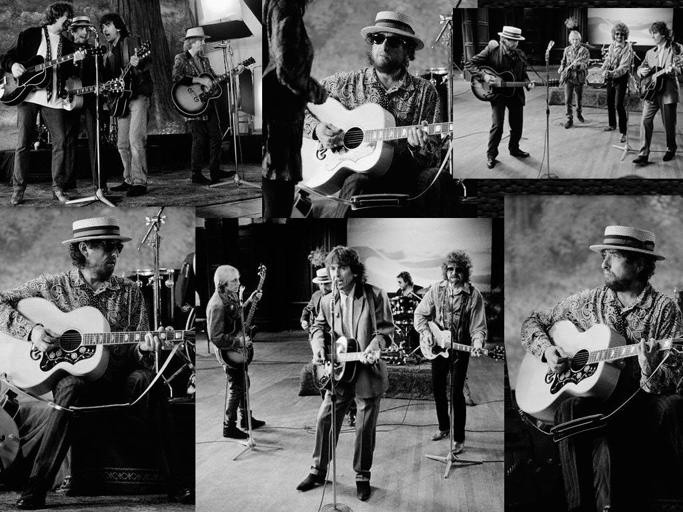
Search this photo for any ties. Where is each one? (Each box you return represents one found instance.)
[84,289,98,309]
[376,87,391,112]
[614,306,627,341]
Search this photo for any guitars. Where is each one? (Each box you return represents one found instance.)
[50,77,125,111]
[471,66,559,101]
[420,321,504,361]
[300,96,453,194]
[559,55,587,85]
[216,264,267,369]
[105,40,152,119]
[312,336,407,390]
[171,56,256,118]
[1,45,107,106]
[515,319,683,423]
[640,65,674,100]
[8,298,196,397]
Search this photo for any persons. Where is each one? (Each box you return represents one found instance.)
[600,23,632,141]
[263,1,327,218]
[522,224,683,512]
[557,30,590,128]
[173,26,235,187]
[633,21,683,164]
[304,10,444,219]
[206,263,268,438]
[2,218,173,510]
[465,26,535,167]
[1,2,153,205]
[295,244,489,501]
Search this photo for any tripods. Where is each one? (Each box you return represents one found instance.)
[612,50,641,162]
[209,56,262,190]
[383,325,422,365]
[232,305,283,461]
[540,55,560,180]
[65,38,122,207]
[425,296,483,479]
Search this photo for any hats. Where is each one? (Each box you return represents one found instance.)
[359,9,425,51]
[61,216,131,244]
[496,25,525,41]
[311,266,333,284]
[177,26,210,39]
[588,225,664,259]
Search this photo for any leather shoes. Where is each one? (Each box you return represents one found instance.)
[128,187,147,196]
[603,125,614,130]
[51,191,75,205]
[240,417,264,430]
[191,175,215,186]
[165,476,195,501]
[452,442,463,455]
[15,482,49,510]
[354,481,371,501]
[210,170,235,180]
[619,134,627,143]
[563,120,571,128]
[511,151,527,157]
[8,191,24,205]
[297,474,325,490]
[630,155,649,165]
[487,159,494,167]
[575,113,583,123]
[223,429,247,439]
[109,183,130,192]
[432,430,448,442]
[661,149,675,162]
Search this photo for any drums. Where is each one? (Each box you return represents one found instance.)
[124,269,187,331]
[391,297,414,324]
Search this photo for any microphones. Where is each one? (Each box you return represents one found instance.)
[224,40,233,54]
[628,39,632,52]
[330,280,337,302]
[239,285,245,301]
[545,40,555,56]
[88,25,99,38]
[450,278,457,295]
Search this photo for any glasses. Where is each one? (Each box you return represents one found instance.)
[366,35,407,45]
[445,266,467,271]
